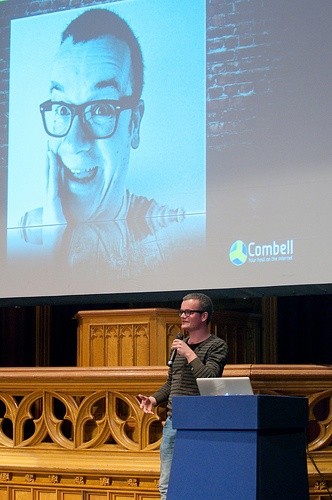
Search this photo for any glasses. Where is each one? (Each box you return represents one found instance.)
[40,100,136,139]
[178,309,205,318]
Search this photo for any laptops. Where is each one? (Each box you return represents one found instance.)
[196,376,254,396]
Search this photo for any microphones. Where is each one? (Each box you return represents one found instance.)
[167,334,183,367]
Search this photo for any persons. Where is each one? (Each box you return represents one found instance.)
[6,8,184,277]
[138,293,229,500]
